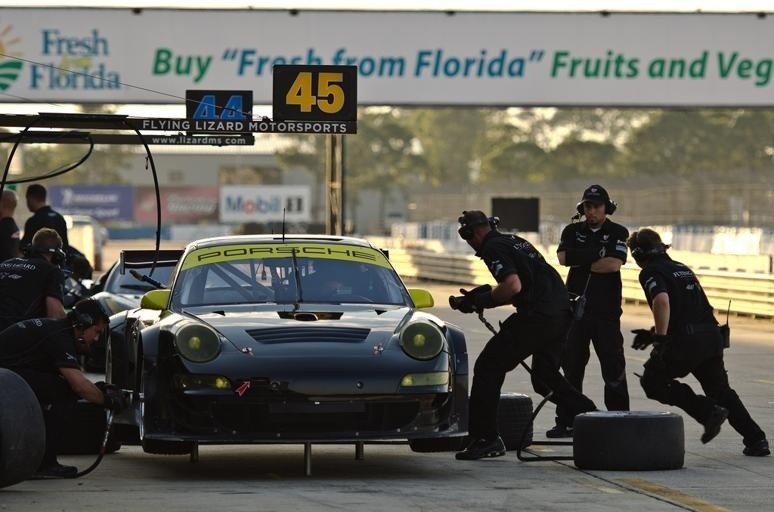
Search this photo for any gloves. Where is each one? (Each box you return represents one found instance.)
[650,332,669,369]
[94,380,129,413]
[630,328,654,351]
[457,284,496,313]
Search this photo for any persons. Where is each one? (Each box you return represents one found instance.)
[0,296,128,477]
[0,226,69,333]
[448,207,601,461]
[319,277,346,302]
[543,182,631,439]
[624,227,771,458]
[19,183,68,257]
[0,190,23,263]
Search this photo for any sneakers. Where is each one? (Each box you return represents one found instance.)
[35,463,78,476]
[545,416,575,439]
[699,404,730,444]
[455,432,508,461]
[743,438,771,457]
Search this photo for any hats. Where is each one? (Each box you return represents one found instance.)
[578,184,610,206]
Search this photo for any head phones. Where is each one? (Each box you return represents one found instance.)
[576,185,618,215]
[457,210,501,240]
[631,232,645,262]
[72,306,95,329]
[23,243,67,266]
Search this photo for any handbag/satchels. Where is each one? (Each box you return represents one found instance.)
[568,291,587,322]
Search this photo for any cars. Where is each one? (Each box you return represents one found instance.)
[63,214,108,270]
